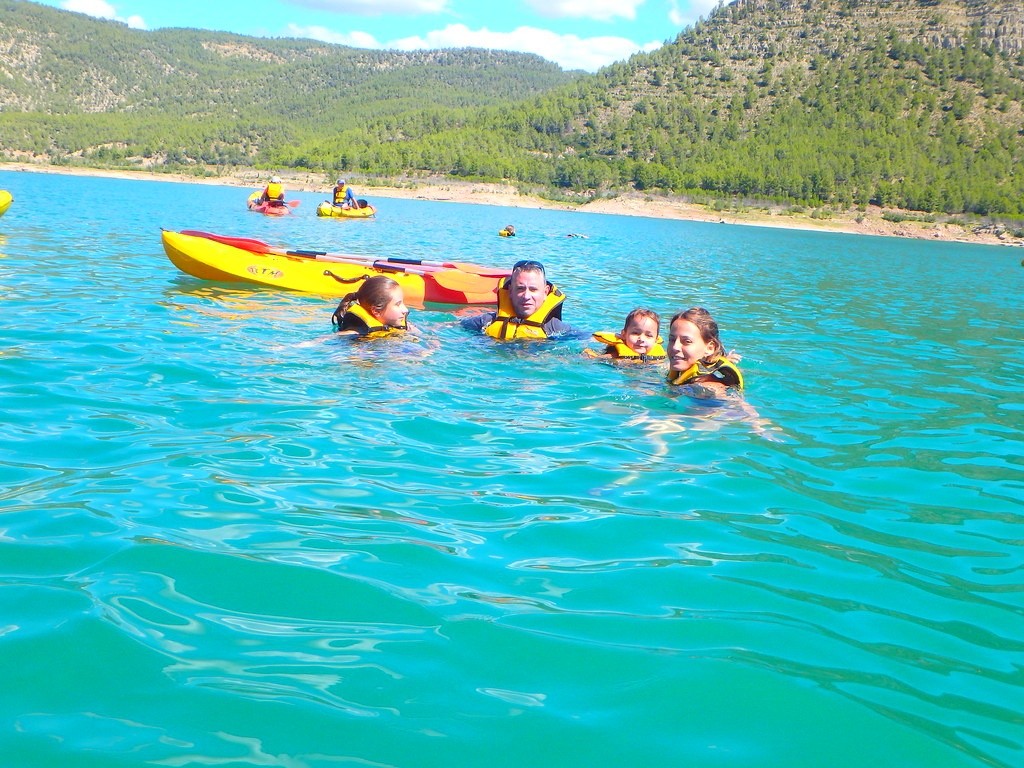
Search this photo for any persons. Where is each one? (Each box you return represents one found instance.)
[664,308,743,387]
[591,308,676,363]
[257,176,285,208]
[333,180,360,211]
[332,276,408,334]
[500,225,516,238]
[458,261,570,339]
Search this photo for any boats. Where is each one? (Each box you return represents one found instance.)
[0,188,15,219]
[158,226,514,306]
[315,199,378,218]
[247,189,291,217]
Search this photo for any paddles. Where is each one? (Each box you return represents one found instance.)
[180,226,514,295]
[324,198,348,209]
[264,198,301,208]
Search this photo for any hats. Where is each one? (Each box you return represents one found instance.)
[336,179,345,184]
[270,176,281,183]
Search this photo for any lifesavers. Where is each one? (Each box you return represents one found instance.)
[592,330,668,359]
